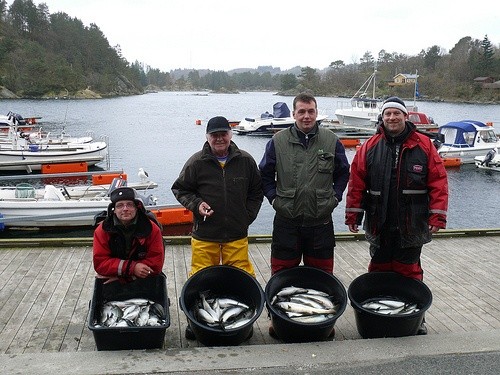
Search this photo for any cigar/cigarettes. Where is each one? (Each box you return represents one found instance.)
[149,270,150,274]
[204,210,208,221]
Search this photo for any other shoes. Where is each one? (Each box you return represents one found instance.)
[184,325,197,340]
[418,323,428,335]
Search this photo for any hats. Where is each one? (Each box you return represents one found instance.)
[107,186,146,216]
[380,97,408,116]
[206,116,232,134]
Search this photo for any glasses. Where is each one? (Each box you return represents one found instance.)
[115,202,135,209]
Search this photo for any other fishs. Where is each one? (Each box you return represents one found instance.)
[98,297,164,326]
[270,284,336,323]
[194,291,253,330]
[362,297,424,316]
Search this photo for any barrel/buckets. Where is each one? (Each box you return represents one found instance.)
[178,264,266,348]
[348,271,433,339]
[265,266,347,343]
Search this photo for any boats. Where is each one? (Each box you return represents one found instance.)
[474,139,500,172]
[0,109,194,233]
[231,64,440,148]
[432,120,500,168]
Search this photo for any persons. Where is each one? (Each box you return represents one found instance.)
[258,92,351,274]
[345,97,449,335]
[92,186,165,285]
[171,115,264,278]
